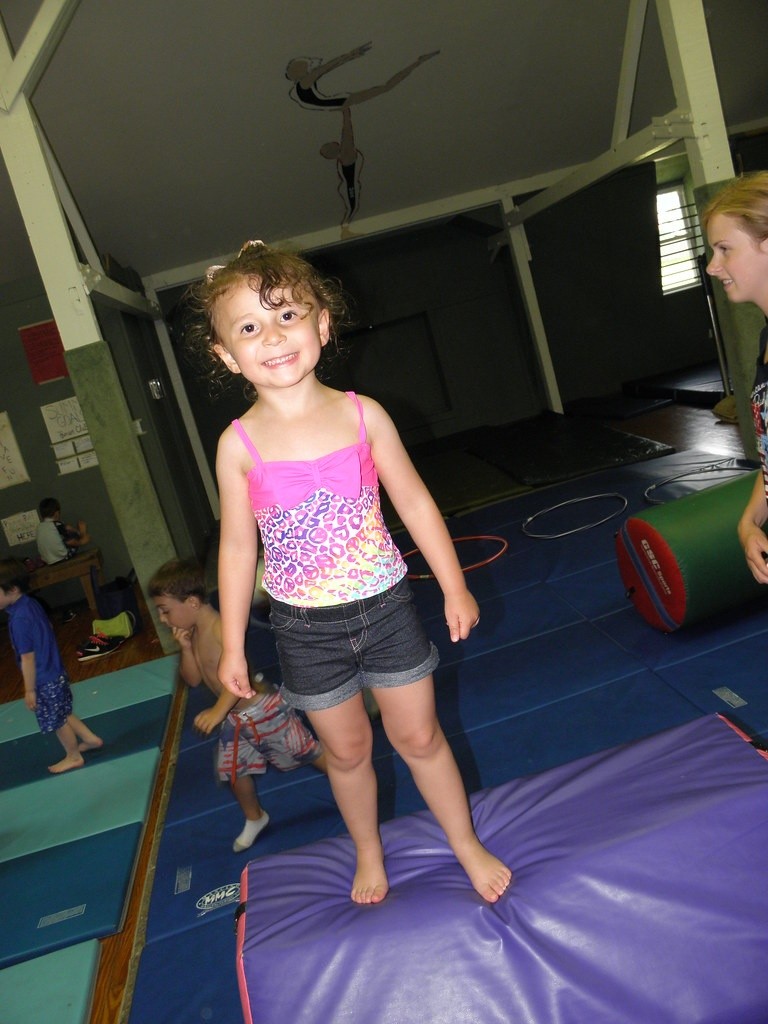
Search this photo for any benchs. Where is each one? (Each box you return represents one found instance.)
[14,546,100,629]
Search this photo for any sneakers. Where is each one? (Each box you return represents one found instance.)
[23,555,44,572]
[62,606,79,623]
[76,632,127,662]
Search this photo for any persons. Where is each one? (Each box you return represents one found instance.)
[1,557,102,773]
[702,170,768,587]
[35,496,90,565]
[147,560,328,852]
[199,241,513,903]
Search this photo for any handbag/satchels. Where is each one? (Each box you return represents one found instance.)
[91,611,137,639]
